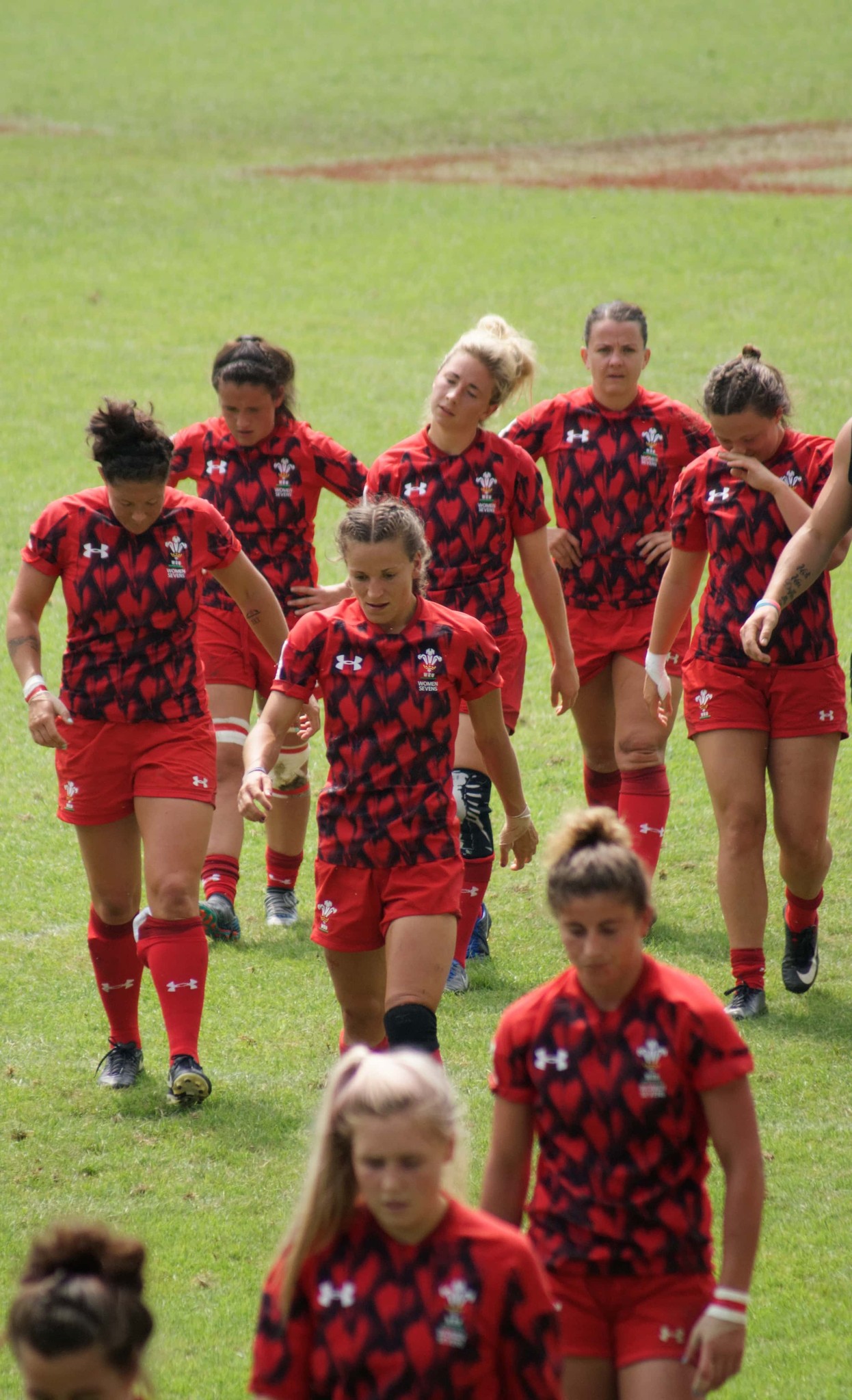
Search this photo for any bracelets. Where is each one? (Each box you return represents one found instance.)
[23,675,49,703]
[704,1289,748,1326]
[242,767,266,785]
[755,599,781,618]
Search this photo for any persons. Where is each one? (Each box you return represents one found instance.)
[362,314,579,995]
[474,806,765,1400]
[7,1220,154,1400]
[237,500,540,1050]
[9,399,318,1105]
[502,303,718,943]
[246,1046,564,1400]
[165,337,370,943]
[645,346,852,1023]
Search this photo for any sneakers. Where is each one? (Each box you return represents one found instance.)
[781,901,818,994]
[722,976,768,1021]
[169,1054,212,1108]
[442,960,471,996]
[198,893,241,942]
[263,887,299,927]
[466,902,491,960]
[93,1036,144,1087]
